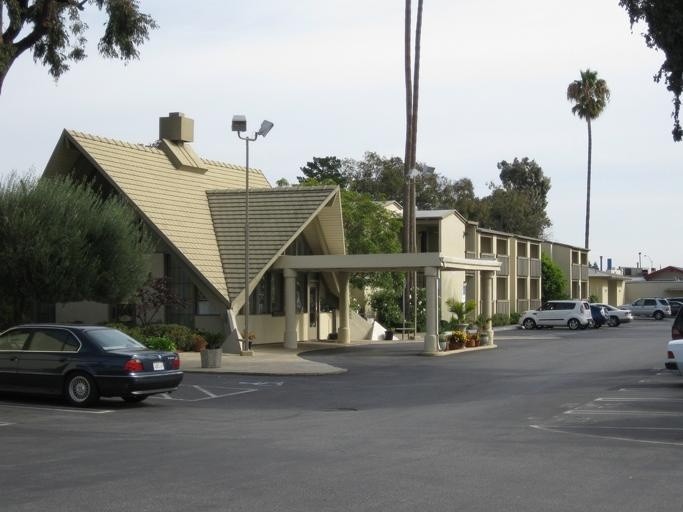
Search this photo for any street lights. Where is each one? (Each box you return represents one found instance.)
[638,252,642,268]
[643,254,654,267]
[228,114,276,356]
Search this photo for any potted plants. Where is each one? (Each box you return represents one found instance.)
[383,301,402,340]
[240,330,256,350]
[439,295,497,351]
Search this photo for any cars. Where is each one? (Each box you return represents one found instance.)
[0,322,184,410]
[516,296,682,376]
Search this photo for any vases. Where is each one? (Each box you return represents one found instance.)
[329,333,338,341]
